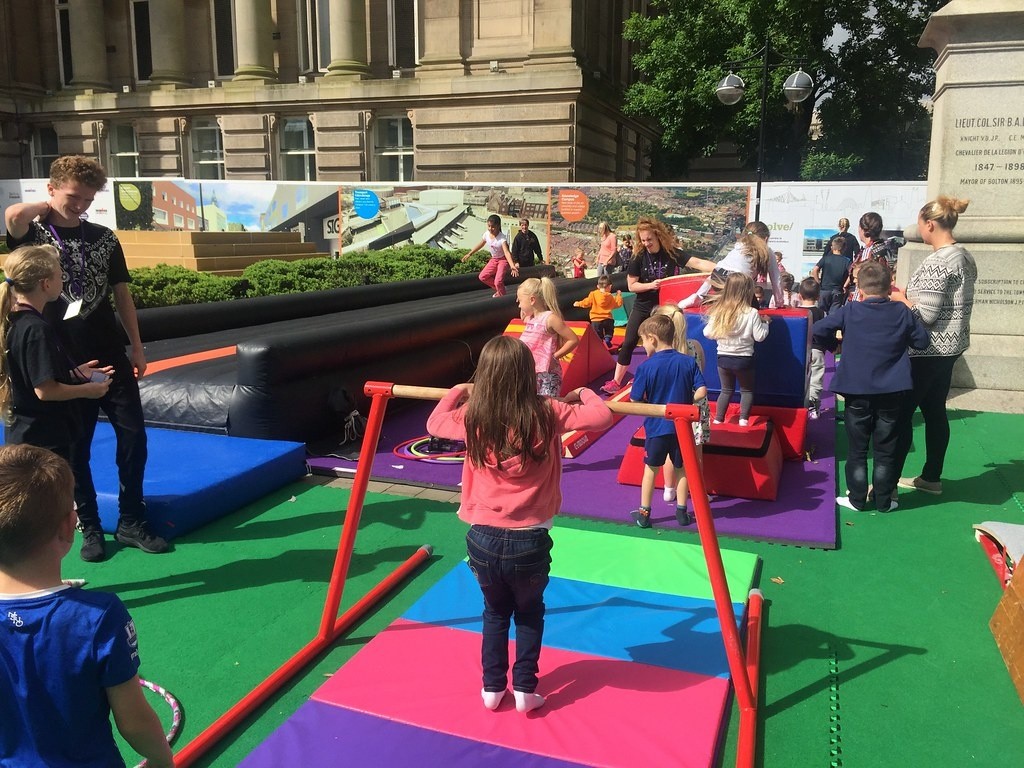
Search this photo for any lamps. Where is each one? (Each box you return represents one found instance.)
[298,75,309,84]
[489,61,499,74]
[392,69,402,79]
[207,80,218,88]
[123,85,133,92]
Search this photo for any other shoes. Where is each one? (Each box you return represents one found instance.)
[898,475,943,496]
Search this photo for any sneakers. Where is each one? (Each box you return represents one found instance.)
[600,380,624,394]
[114,519,168,553]
[80,526,105,561]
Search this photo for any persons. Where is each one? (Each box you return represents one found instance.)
[0,443,175,768]
[796,277,826,418]
[846,195,977,501]
[426,335,615,712]
[572,253,586,278]
[703,271,772,427]
[600,218,716,394]
[596,223,617,275]
[461,215,519,298]
[618,234,633,270]
[752,212,903,316]
[710,221,786,309]
[512,219,545,269]
[650,304,713,502]
[812,263,931,513]
[573,276,623,347]
[0,244,116,456]
[630,315,707,529]
[516,277,579,398]
[4,155,169,563]
